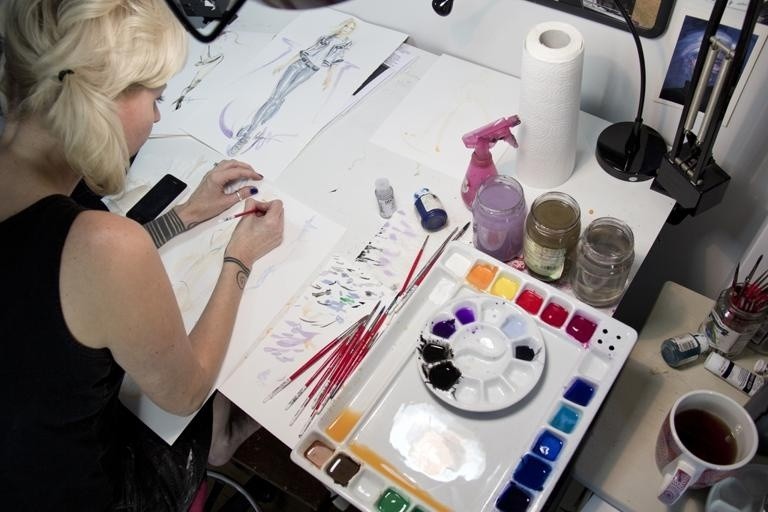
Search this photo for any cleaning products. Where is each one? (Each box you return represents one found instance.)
[463,116,519,210]
[519,22,584,186]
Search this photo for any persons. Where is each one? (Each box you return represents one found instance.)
[1,1,286,511]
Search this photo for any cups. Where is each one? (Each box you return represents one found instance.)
[651,386,759,508]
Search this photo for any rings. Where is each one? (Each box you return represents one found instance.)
[235,191,242,202]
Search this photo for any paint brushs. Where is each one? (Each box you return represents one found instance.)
[389,221,469,330]
[705,255,768,358]
[226,208,259,221]
[263,298,390,438]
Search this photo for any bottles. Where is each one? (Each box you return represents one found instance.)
[703,350,766,396]
[574,216,638,310]
[661,331,711,368]
[698,280,768,360]
[470,172,527,261]
[522,190,583,282]
